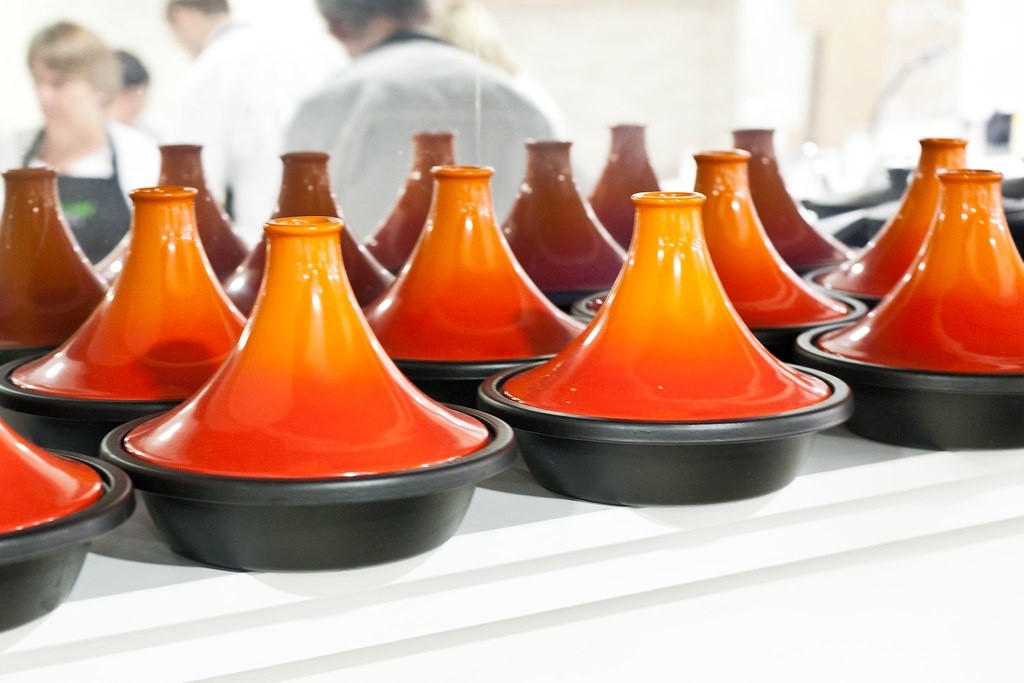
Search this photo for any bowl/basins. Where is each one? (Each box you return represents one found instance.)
[1,128,1024,634]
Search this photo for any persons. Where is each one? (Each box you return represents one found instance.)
[104,51,151,125]
[0,23,163,265]
[170,0,268,225]
[279,0,555,246]
